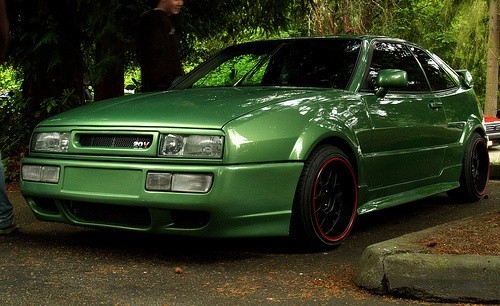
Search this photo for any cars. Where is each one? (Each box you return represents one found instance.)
[18,35,490,248]
[485,114,500,151]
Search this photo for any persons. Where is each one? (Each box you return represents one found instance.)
[0,149,16,234]
[136,0,186,92]
